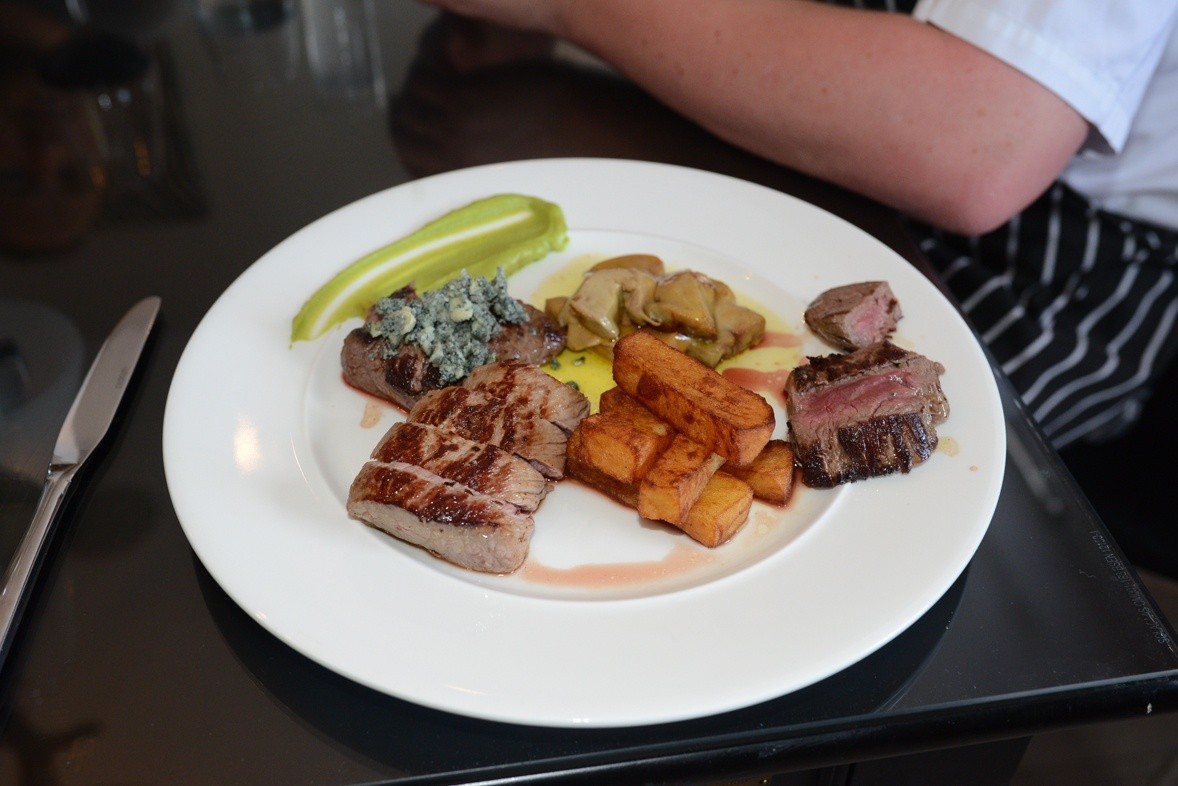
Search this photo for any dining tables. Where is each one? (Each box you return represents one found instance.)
[0,0,1178,786]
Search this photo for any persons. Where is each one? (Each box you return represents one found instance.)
[420,0,1178,786]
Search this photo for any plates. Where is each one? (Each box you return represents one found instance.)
[161,159,1005,729]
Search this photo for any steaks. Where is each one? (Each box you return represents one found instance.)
[784,281,952,488]
[340,277,593,574]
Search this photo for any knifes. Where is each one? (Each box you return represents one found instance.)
[0,294,161,654]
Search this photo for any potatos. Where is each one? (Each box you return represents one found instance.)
[566,329,795,550]
